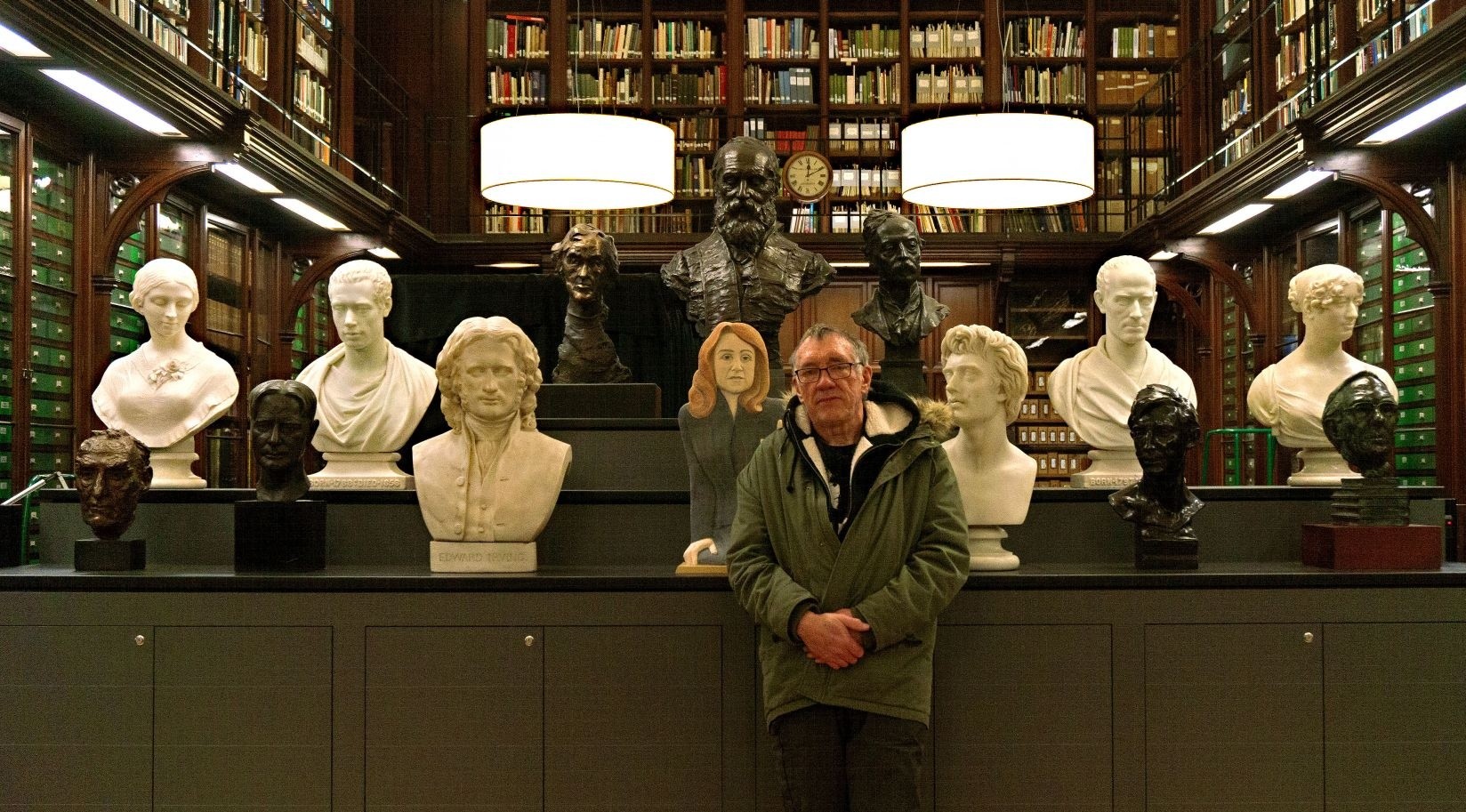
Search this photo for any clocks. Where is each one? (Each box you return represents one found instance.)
[783,151,833,202]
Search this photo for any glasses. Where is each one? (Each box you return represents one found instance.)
[795,363,863,383]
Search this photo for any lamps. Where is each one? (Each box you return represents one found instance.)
[480,0,675,210]
[901,0,1094,208]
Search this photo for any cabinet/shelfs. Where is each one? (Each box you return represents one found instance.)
[0,0,1466,489]
[0,586,1466,812]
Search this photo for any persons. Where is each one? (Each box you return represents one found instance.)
[74,429,153,540]
[727,325,972,812]
[1107,383,1206,537]
[91,258,240,453]
[661,135,837,369]
[246,380,320,505]
[850,208,951,359]
[939,325,1038,526]
[550,224,633,383]
[296,259,439,454]
[1047,255,1198,449]
[678,322,789,566]
[411,316,574,544]
[1247,263,1403,479]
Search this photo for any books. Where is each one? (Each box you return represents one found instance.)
[1215,0,1432,168]
[110,0,336,165]
[484,10,1179,235]
